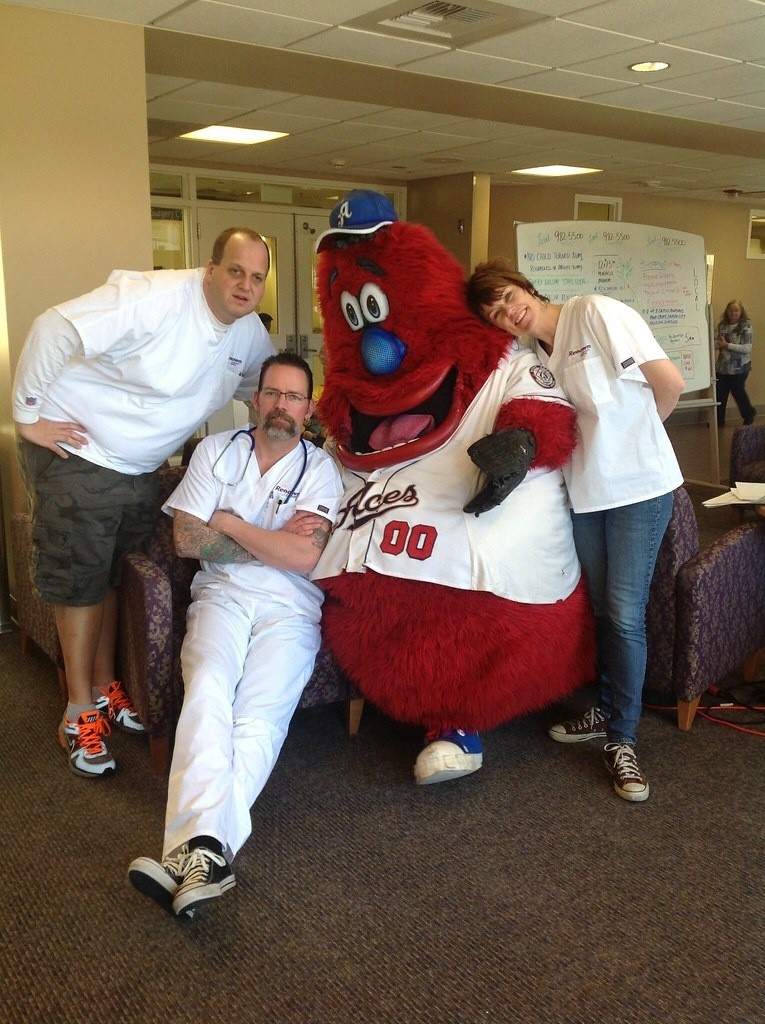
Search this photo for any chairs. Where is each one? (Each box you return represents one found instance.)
[729,424,765,524]
[123,463,367,773]
[8,513,69,703]
[588,487,765,732]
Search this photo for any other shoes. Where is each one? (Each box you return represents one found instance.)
[743,408,757,425]
[708,420,726,427]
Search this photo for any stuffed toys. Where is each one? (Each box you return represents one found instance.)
[309,189,601,784]
[302,399,329,440]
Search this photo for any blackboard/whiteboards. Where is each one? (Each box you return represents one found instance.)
[513,220,713,394]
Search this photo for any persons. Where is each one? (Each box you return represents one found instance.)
[243,313,273,429]
[12,227,280,776]
[707,300,757,428]
[464,256,684,802]
[128,353,345,920]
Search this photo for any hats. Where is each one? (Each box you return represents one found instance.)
[259,313,274,321]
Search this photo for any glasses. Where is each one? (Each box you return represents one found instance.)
[261,390,311,404]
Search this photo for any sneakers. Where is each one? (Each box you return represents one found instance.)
[547,705,609,743]
[602,741,649,802]
[127,855,199,921]
[90,680,147,735]
[56,708,117,778]
[172,848,238,915]
